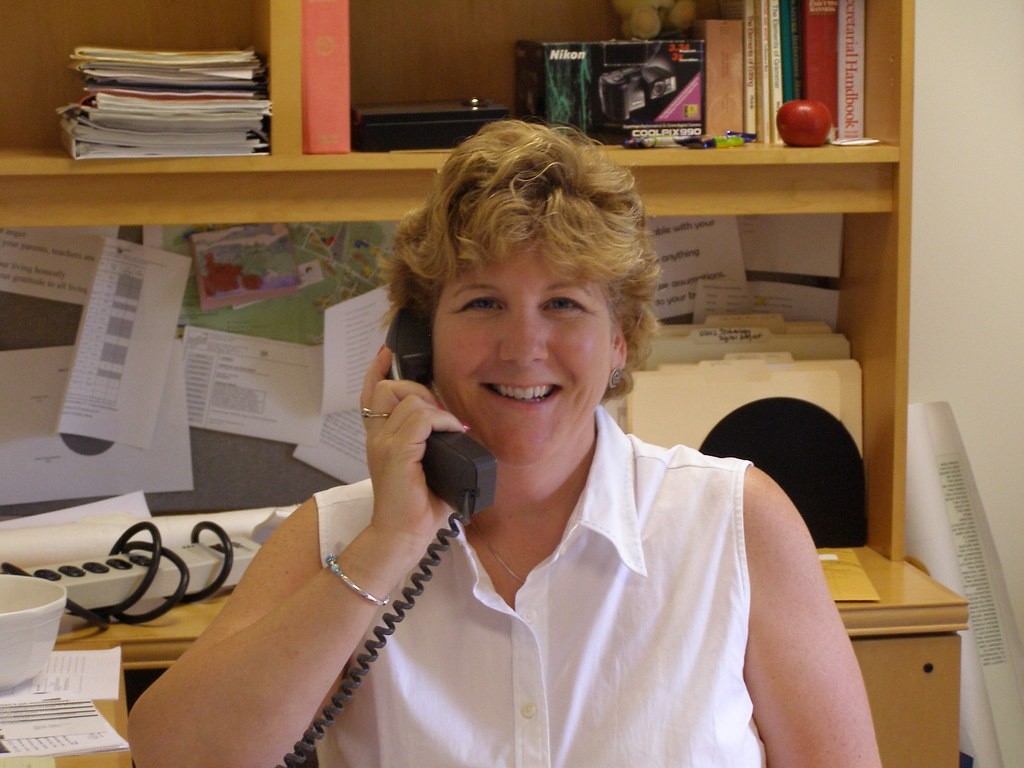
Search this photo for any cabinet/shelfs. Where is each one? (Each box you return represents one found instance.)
[0,0,918,564]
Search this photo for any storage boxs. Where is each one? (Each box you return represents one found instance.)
[589,39,704,136]
[513,38,594,133]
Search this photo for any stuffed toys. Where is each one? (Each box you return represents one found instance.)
[613,0,696,39]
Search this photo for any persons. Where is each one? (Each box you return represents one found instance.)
[128,120,883,768]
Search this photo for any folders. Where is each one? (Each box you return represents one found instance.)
[58,126,270,161]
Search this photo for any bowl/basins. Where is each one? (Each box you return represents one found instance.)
[0,572,68,692]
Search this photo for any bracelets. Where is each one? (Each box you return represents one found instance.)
[326,554,389,606]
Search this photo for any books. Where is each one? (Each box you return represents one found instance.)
[699,0,865,144]
[58,48,269,160]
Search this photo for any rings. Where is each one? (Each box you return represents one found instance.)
[360,407,390,418]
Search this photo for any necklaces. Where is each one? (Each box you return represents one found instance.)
[471,520,525,584]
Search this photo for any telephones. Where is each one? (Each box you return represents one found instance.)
[382,307,496,515]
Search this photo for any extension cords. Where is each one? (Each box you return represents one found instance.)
[8,534,261,613]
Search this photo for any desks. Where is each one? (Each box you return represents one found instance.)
[53,544,971,768]
[0,638,134,768]
